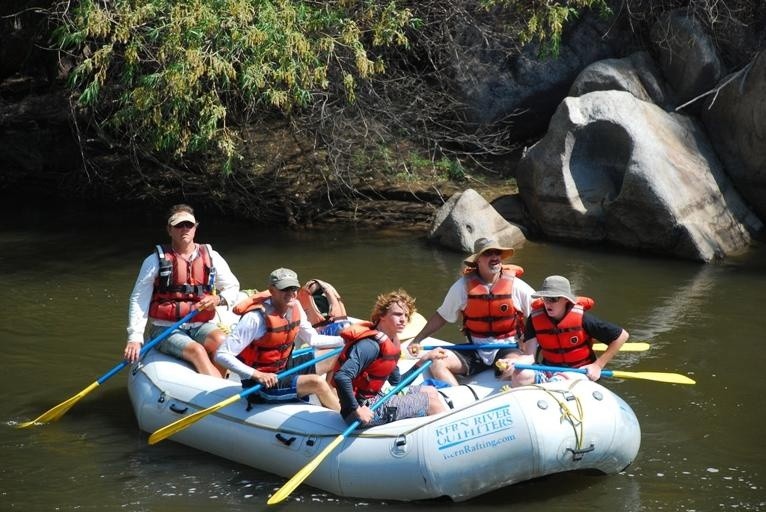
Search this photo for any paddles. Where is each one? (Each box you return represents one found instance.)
[496,361,696,385]
[267,360,433,505]
[412,343,649,352]
[149,347,344,445]
[19,306,204,429]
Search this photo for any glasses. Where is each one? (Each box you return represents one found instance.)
[282,286,297,292]
[481,249,502,256]
[541,296,560,302]
[175,221,193,228]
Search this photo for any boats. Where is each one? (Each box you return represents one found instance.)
[123,286,646,504]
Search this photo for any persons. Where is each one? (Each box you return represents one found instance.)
[499,275,630,386]
[406,237,541,388]
[215,267,341,410]
[123,205,240,378]
[330,289,450,430]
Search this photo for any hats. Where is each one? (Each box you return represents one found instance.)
[531,276,575,305]
[464,237,514,267]
[169,211,197,228]
[270,267,301,290]
